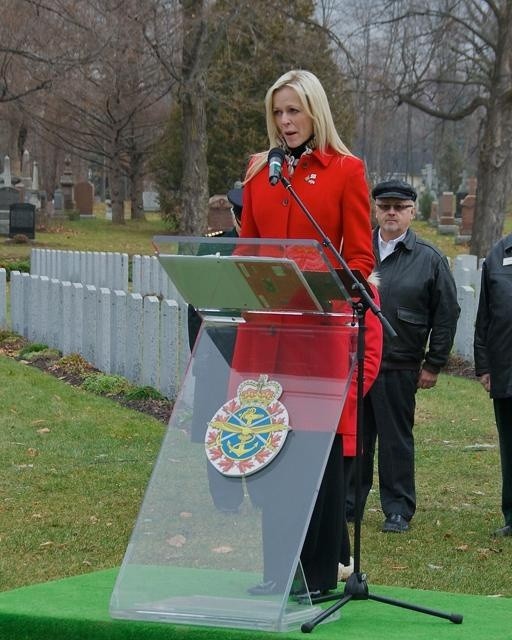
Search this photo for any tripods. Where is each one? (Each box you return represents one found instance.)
[298,302,463,634]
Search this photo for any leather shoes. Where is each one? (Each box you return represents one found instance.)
[248,578,293,599]
[295,586,332,600]
[382,513,409,532]
[491,525,512,537]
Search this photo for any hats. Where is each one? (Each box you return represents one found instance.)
[227,188,243,228]
[372,179,417,202]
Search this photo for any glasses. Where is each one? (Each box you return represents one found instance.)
[375,204,413,210]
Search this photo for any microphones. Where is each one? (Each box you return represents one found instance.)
[268,147,284,186]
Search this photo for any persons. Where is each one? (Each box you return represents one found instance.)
[351,179,461,533]
[472,234,511,537]
[187,186,263,516]
[228,69,379,605]
[337,272,385,583]
